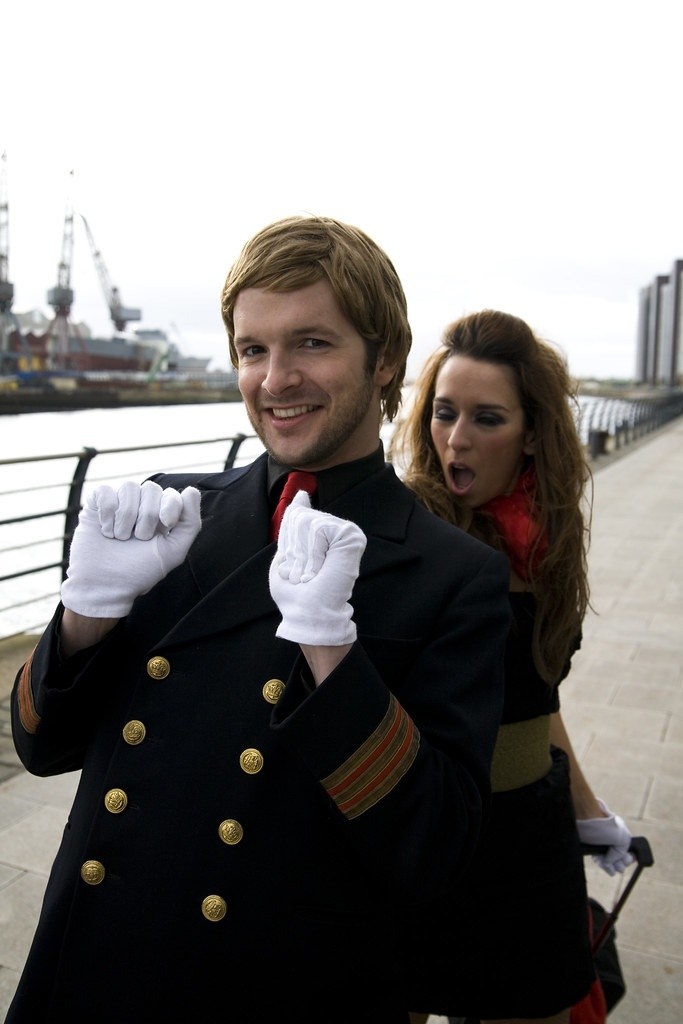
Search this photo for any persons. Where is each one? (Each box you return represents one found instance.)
[0,214,603,1021]
[403,314,636,877]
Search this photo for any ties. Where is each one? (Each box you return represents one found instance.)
[272,471,316,542]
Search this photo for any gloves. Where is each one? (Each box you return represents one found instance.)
[268,490,368,646]
[61,476,202,619]
[576,797,637,875]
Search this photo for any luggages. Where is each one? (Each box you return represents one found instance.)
[573,837,658,1019]
